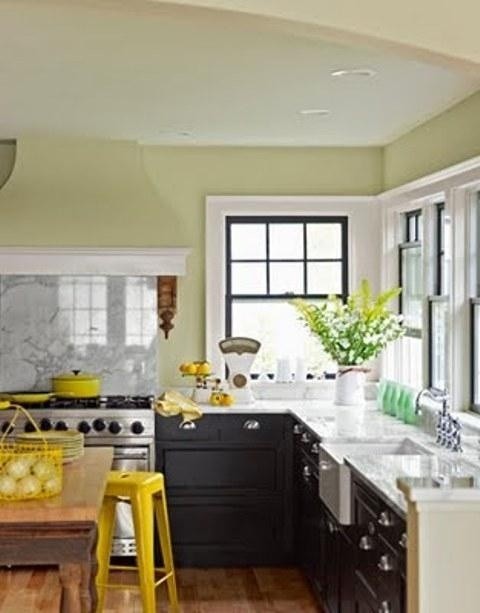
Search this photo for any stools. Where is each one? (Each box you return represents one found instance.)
[94,467,182,611]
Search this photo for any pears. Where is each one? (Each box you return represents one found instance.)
[0,457,62,500]
[179,360,234,407]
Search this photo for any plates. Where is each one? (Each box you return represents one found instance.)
[16,430,85,465]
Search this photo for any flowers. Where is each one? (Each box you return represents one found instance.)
[285,276,413,374]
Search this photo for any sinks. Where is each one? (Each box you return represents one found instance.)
[316,431,438,527]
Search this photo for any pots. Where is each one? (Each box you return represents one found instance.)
[0,384,79,403]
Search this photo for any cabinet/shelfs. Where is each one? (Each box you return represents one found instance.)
[155,414,293,570]
[330,469,408,611]
[290,419,330,611]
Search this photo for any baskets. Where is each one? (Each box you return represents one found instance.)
[0,404,64,501]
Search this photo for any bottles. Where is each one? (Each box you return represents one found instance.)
[376,377,423,426]
[255,354,328,399]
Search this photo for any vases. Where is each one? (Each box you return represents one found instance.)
[332,365,366,406]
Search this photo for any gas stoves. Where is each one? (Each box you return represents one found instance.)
[0,393,155,446]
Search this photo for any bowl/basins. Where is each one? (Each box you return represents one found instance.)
[0,399,11,410]
[45,368,102,399]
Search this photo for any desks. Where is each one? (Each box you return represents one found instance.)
[0,441,116,610]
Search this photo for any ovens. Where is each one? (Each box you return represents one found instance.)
[113,445,154,557]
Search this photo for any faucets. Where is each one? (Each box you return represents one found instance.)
[412,385,454,445]
